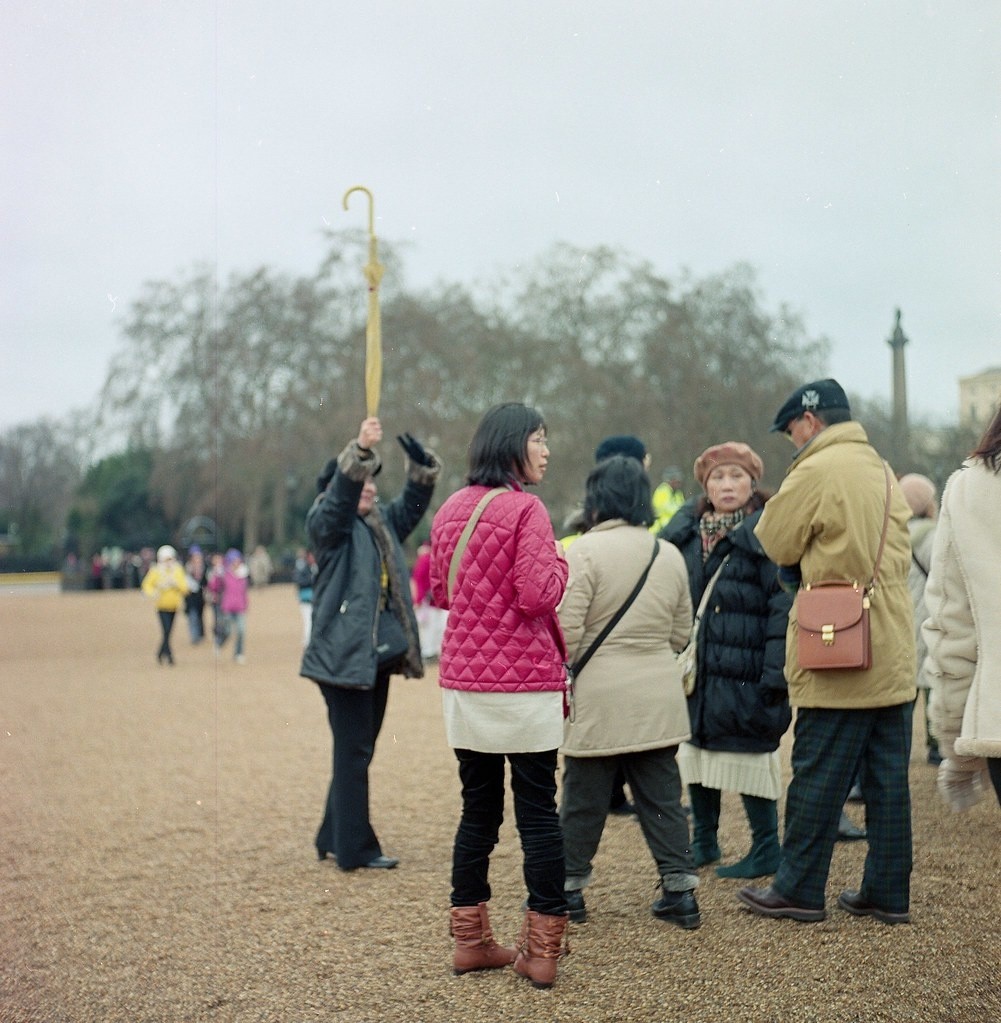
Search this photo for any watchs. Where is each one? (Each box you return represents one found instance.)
[355,442,369,452]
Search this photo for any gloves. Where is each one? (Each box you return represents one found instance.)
[936,768,990,816]
[397,432,426,465]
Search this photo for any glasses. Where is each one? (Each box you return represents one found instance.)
[780,414,800,443]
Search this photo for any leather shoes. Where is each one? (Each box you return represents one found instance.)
[736,883,826,922]
[838,889,910,924]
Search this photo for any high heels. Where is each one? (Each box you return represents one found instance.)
[365,855,399,869]
[318,847,335,860]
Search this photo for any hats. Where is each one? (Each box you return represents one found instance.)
[693,440,764,492]
[768,378,850,434]
[316,460,382,493]
[897,473,935,518]
[225,546,243,565]
[156,545,177,561]
[186,543,201,557]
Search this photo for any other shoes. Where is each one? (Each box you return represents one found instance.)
[836,825,867,841]
[156,654,177,668]
[845,789,866,804]
[928,748,942,765]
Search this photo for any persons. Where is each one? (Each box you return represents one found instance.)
[58,535,319,669]
[916,404,1001,812]
[739,378,924,924]
[300,418,442,867]
[555,454,706,931]
[429,403,571,988]
[557,435,651,538]
[656,438,801,882]
[407,534,447,668]
[898,471,943,766]
[643,464,686,534]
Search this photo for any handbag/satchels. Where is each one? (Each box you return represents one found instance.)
[376,608,410,668]
[795,579,873,672]
[674,635,697,697]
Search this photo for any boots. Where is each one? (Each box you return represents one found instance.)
[689,783,721,870]
[714,792,782,879]
[650,873,700,930]
[449,902,517,976]
[522,875,589,923]
[512,905,570,989]
